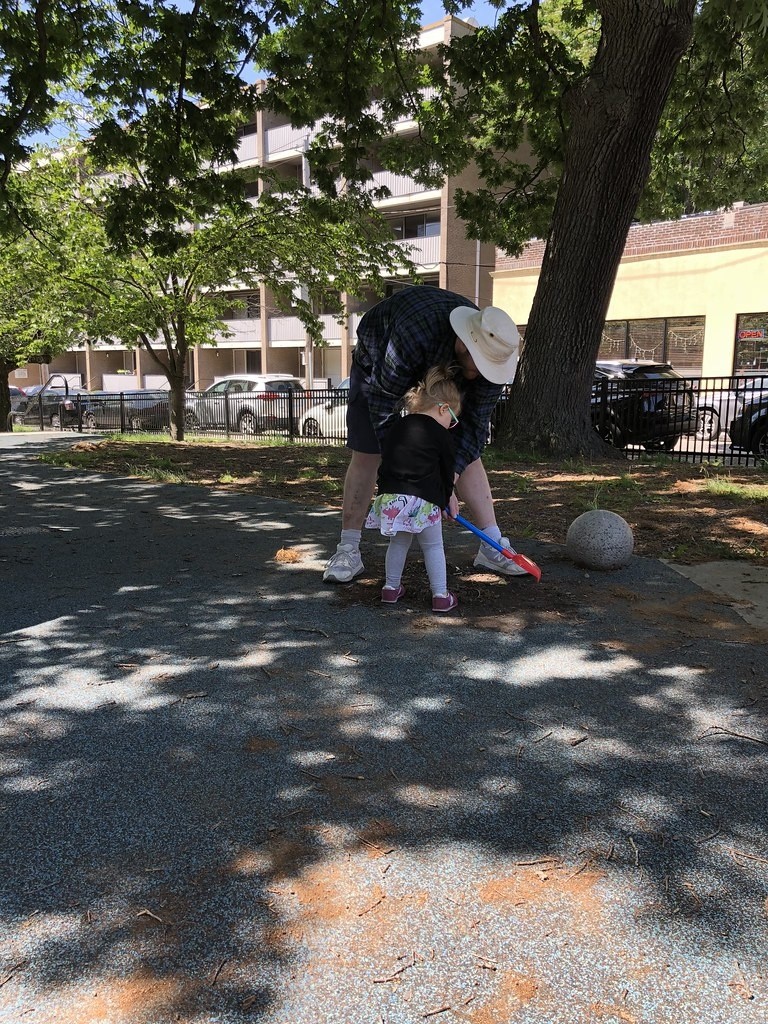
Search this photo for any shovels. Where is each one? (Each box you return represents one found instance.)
[445,506,541,584]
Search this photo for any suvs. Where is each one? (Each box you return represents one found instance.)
[489,359,702,454]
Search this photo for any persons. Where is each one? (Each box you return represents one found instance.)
[322,286,530,582]
[366,366,458,612]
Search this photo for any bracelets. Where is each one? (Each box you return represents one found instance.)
[452,483,458,493]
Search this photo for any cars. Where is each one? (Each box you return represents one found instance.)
[12,386,104,428]
[729,392,768,463]
[296,377,350,440]
[81,389,170,431]
[694,375,768,442]
[184,374,306,430]
[8,386,32,408]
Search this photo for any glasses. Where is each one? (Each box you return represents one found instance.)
[439,403,459,428]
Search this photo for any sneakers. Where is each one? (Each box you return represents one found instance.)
[432,592,458,611]
[323,544,365,582]
[381,584,406,603]
[473,537,529,575]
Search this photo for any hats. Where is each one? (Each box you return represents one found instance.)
[450,306,519,385]
